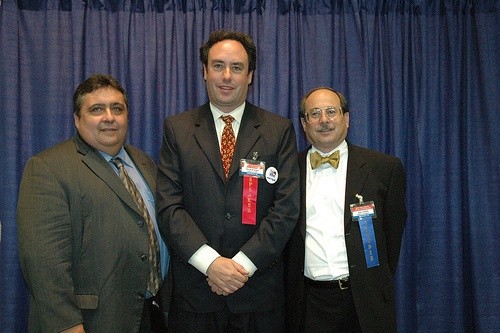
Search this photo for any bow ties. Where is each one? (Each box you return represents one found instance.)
[310,149,341,170]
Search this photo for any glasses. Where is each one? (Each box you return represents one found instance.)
[303,106,343,124]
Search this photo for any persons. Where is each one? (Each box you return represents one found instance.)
[155,31,301,333]
[284,87,409,333]
[17,73,174,333]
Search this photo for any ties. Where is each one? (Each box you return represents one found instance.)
[220,114,237,177]
[109,156,164,298]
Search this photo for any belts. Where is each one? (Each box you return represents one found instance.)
[304,276,351,291]
